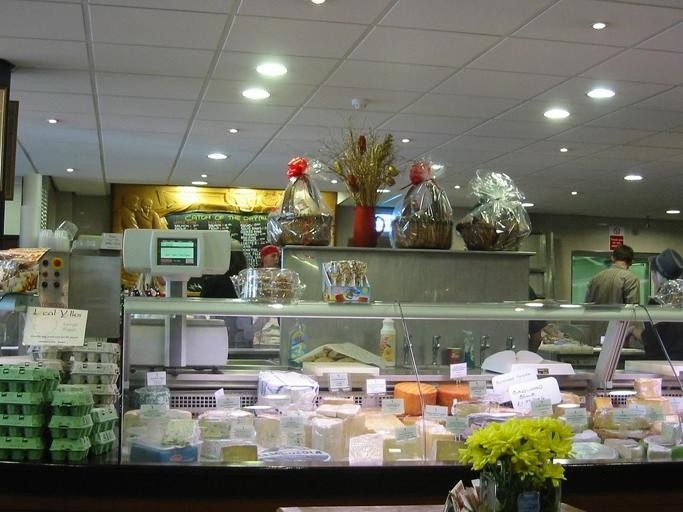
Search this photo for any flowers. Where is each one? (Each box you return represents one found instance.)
[316,108,403,207]
[457,413,574,487]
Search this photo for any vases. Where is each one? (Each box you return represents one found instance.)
[351,205,386,247]
[479,472,562,511]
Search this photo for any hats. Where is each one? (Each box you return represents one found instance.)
[647,248,683,280]
[261,244,280,256]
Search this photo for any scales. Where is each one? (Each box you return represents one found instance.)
[126,229,230,375]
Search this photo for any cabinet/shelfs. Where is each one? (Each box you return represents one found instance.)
[122,299,681,464]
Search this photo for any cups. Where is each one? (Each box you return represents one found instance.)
[448,347,461,364]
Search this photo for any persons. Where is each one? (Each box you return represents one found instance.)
[200,239,247,298]
[528,285,549,353]
[260,245,281,269]
[112,193,140,235]
[627,247,683,363]
[584,244,643,350]
[137,198,167,229]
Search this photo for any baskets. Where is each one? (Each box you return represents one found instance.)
[268,175,333,246]
[391,182,453,249]
[456,198,531,251]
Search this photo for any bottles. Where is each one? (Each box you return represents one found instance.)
[379,318,397,371]
